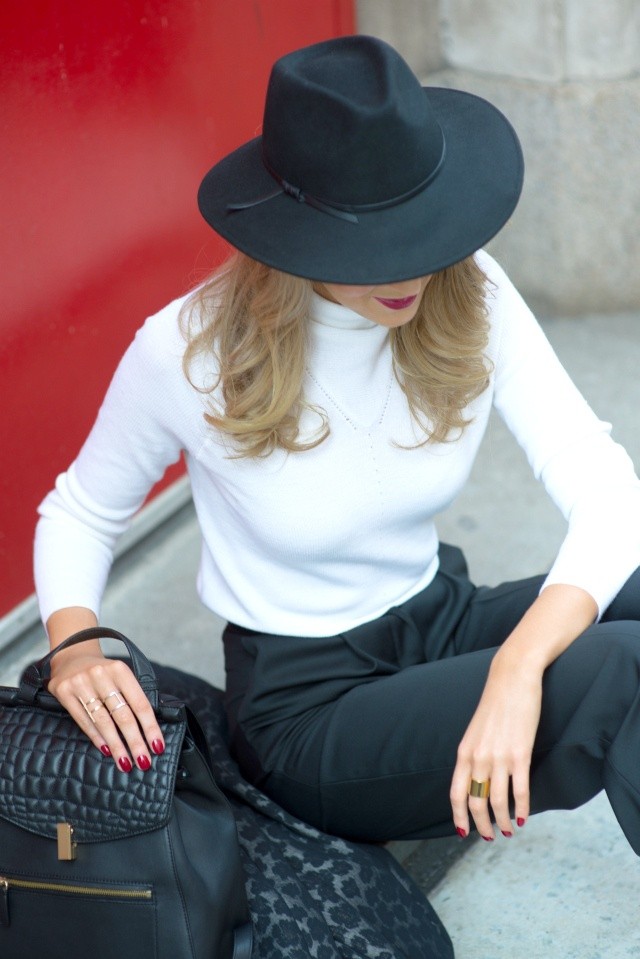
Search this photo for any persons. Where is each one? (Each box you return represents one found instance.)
[33,36,640,858]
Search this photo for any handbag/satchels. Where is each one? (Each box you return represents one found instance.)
[0,627,239,959]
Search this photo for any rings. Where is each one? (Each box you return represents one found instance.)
[78,695,104,723]
[468,778,491,798]
[102,691,127,715]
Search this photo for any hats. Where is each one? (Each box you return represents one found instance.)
[196,35,526,285]
